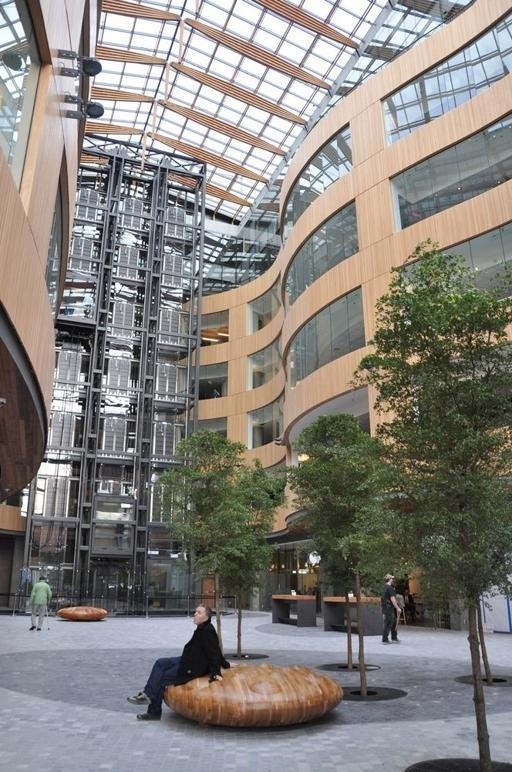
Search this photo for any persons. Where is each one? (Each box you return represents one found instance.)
[402,589,416,624]
[125,603,240,721]
[29,577,53,630]
[380,574,401,644]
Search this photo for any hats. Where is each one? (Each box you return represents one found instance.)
[384,573,394,579]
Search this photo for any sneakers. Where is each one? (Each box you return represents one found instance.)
[136,712,161,721]
[29,626,42,631]
[381,639,402,645]
[127,691,154,705]
